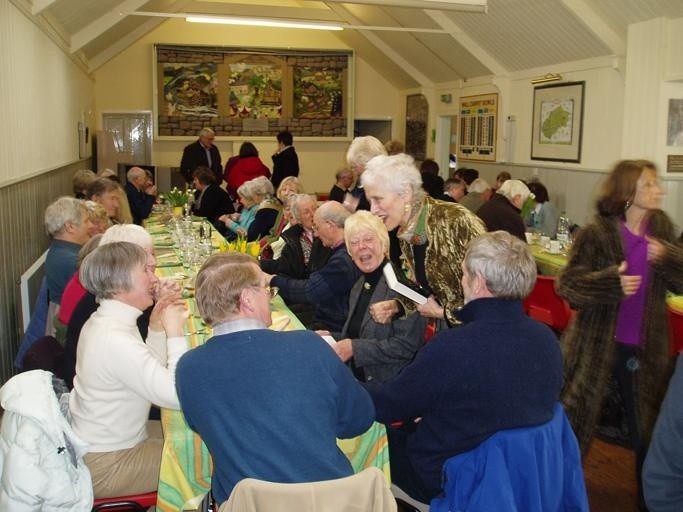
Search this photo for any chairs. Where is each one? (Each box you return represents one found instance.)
[525,270,574,330]
[385,313,440,429]
[211,461,395,512]
[663,297,681,360]
[389,395,573,511]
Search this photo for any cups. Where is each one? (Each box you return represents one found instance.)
[151,203,212,276]
[525,232,573,254]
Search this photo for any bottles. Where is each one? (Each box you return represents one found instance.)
[556,211,569,251]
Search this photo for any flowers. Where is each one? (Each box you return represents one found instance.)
[159,186,199,208]
[221,230,264,261]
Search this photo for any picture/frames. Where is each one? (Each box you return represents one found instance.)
[528,78,587,163]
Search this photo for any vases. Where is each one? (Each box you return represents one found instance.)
[171,206,182,217]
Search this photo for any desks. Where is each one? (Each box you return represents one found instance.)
[518,233,575,273]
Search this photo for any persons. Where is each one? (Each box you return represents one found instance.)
[13,121,553,424]
[356,230,561,512]
[553,159,683,511]
[174,253,375,511]
[69,241,195,500]
[640,351,683,512]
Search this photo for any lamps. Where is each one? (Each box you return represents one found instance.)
[183,11,346,35]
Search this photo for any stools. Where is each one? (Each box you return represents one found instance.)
[92,485,160,510]
